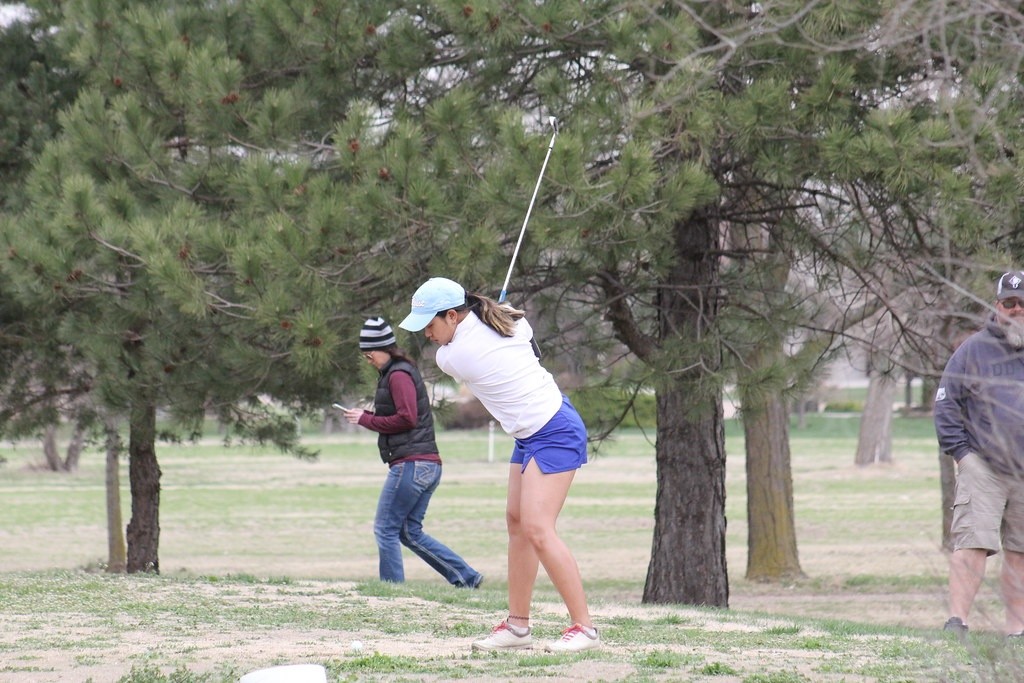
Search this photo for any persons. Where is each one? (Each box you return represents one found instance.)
[399,277,602,647]
[932,268,1024,639]
[341,314,487,589]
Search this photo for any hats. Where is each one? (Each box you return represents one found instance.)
[358,317,396,350]
[996,271,1024,299]
[397,276,466,333]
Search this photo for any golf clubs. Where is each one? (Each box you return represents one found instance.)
[497,113,561,304]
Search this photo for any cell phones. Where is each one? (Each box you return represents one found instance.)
[332,403,349,416]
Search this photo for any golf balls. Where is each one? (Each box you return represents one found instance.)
[351,640,362,651]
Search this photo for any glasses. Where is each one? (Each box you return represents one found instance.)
[361,351,374,360]
[998,296,1024,309]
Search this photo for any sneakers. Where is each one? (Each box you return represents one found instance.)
[543,624,599,654]
[1005,630,1023,641]
[471,620,533,651]
[942,616,969,641]
